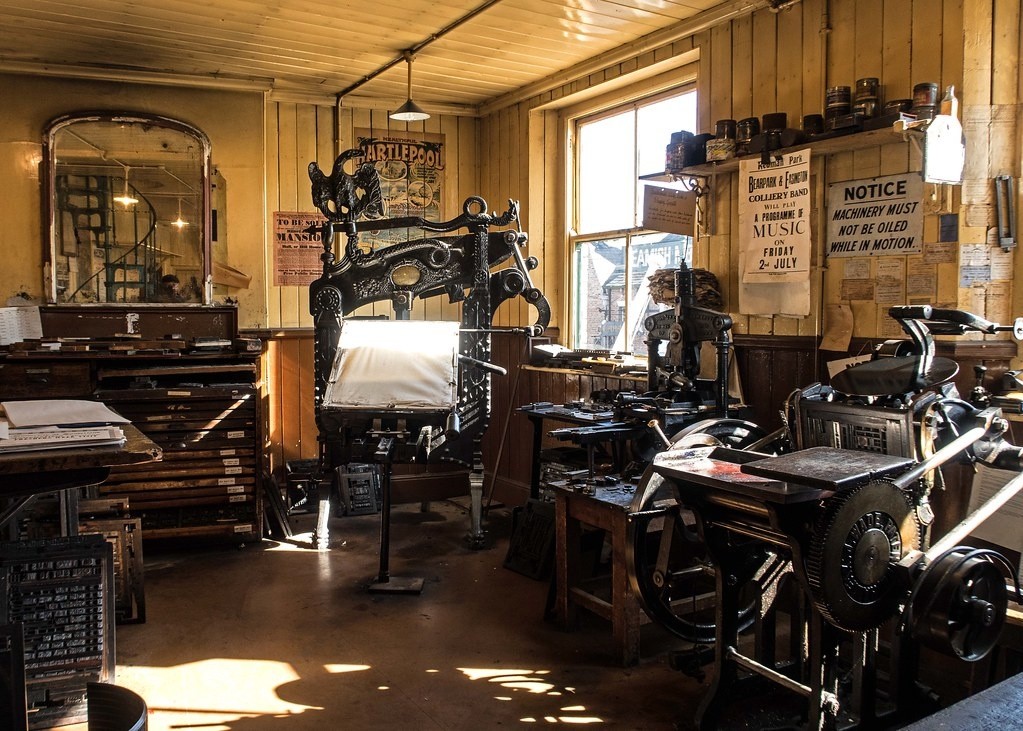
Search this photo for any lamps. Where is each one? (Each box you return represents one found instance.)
[112,161,140,211]
[167,197,191,231]
[387,50,432,122]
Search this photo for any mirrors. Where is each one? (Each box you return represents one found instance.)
[42,109,210,307]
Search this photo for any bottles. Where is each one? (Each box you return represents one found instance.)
[940,84,958,118]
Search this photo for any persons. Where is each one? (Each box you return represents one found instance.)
[159,274,185,303]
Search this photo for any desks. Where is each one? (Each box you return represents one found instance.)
[523,402,658,505]
[0,400,162,537]
[556,458,712,669]
